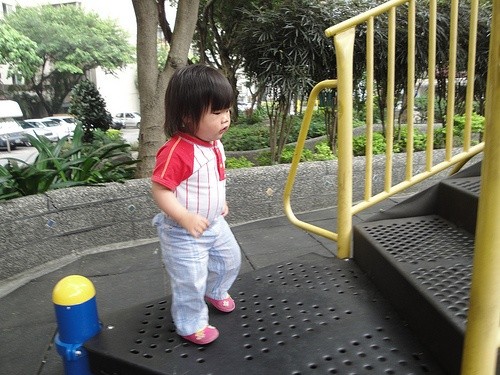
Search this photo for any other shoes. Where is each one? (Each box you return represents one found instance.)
[181,323,220,345]
[205,295,236,313]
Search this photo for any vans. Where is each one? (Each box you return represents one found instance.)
[0,100,27,147]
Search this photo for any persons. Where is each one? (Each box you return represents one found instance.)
[151,62,241,344]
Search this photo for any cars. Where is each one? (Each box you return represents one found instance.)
[113,112,141,128]
[20,116,83,149]
[111,118,122,130]
